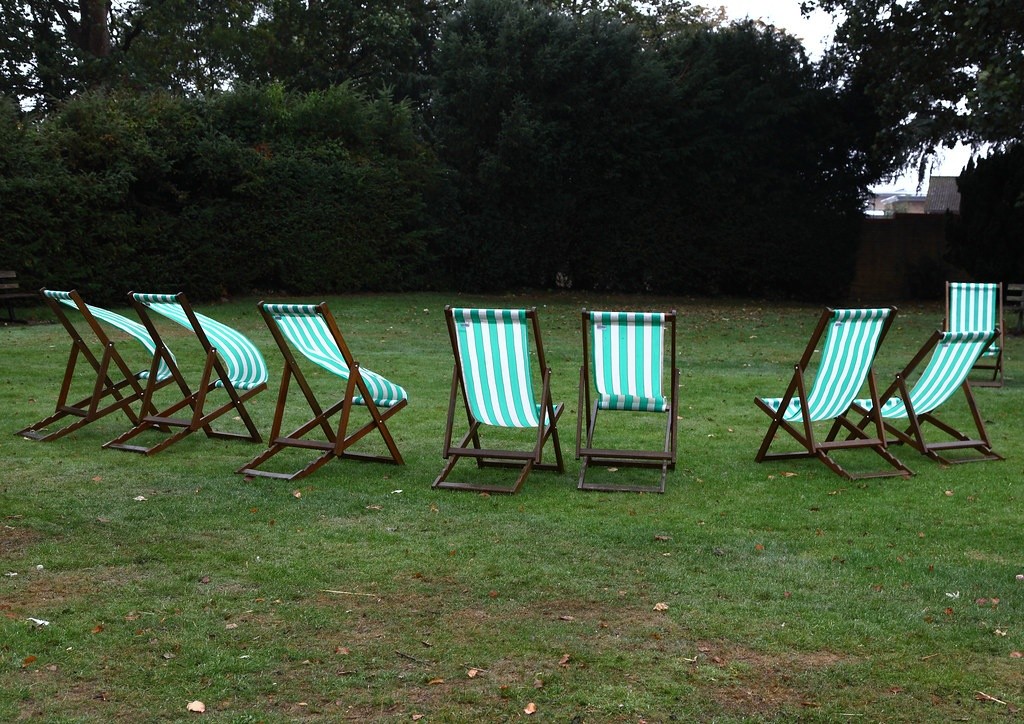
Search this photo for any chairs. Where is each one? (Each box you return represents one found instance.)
[234,300,408,481]
[938,280,1006,387]
[575,308,680,494]
[432,305,564,496]
[845,328,1007,466]
[754,305,916,483]
[13,287,174,444]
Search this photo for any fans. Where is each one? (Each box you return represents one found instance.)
[101,291,267,457]
